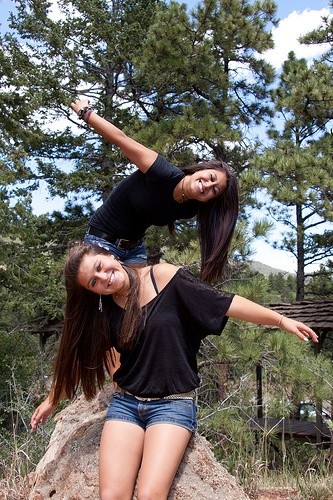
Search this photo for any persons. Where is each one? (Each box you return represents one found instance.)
[69,96,238,392]
[31,242,320,500]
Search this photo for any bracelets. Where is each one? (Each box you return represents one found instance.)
[277,310,286,330]
[77,105,94,126]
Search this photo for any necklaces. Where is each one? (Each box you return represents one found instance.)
[179,175,187,204]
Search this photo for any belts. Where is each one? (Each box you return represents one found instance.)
[114,382,194,402]
[87,224,142,250]
